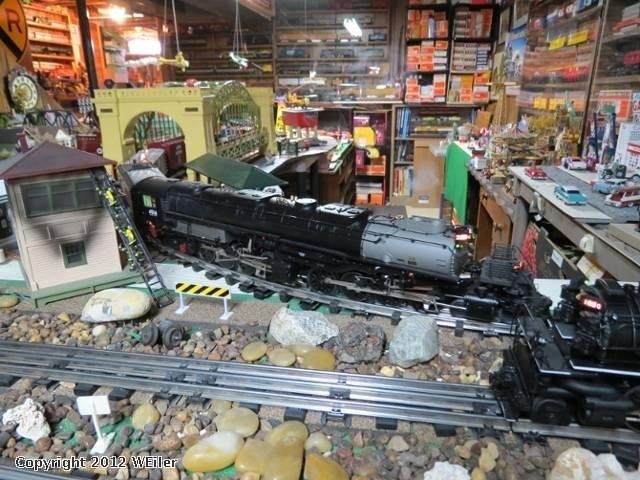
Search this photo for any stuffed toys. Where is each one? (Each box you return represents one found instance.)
[0,78,640,430]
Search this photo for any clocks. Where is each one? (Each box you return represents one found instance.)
[7,67,39,112]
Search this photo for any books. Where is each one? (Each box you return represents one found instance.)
[0,78,640,430]
